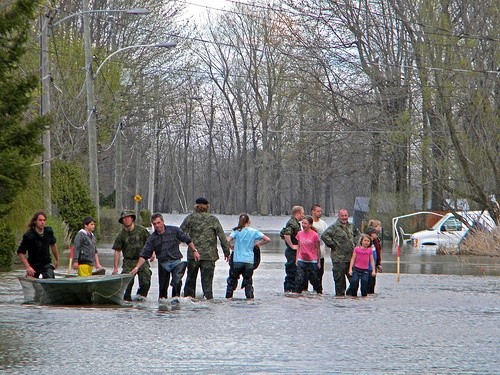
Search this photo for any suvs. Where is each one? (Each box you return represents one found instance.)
[411,210,496,247]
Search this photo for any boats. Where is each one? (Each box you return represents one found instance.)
[16,272,132,306]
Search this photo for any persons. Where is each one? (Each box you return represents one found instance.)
[17,211,60,279]
[280,206,304,292]
[72,216,104,277]
[129,213,201,299]
[226,214,271,299]
[111,209,155,302]
[365,219,383,294]
[311,204,327,291]
[178,197,231,300]
[320,209,362,296]
[346,234,376,297]
[295,216,322,295]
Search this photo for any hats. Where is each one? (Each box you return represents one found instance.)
[196,198,209,204]
[368,219,381,228]
[366,226,375,234]
[118,210,136,223]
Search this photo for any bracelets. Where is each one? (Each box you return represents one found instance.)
[193,249,197,252]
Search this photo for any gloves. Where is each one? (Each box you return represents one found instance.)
[330,243,337,250]
